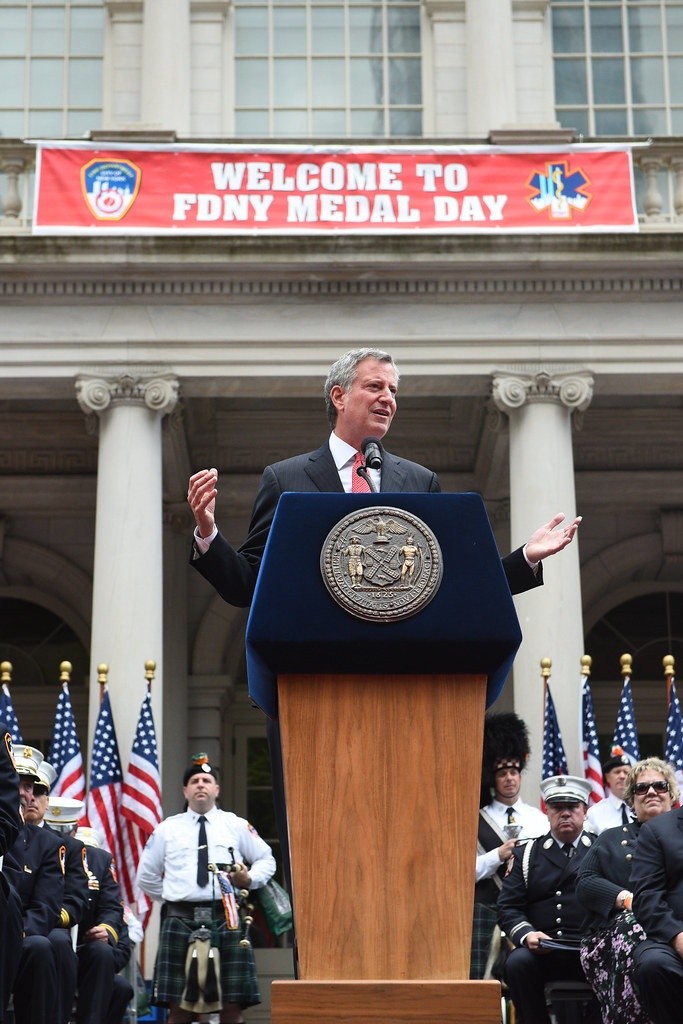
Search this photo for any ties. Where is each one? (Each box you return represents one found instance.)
[197,815,209,888]
[352,451,372,493]
[505,807,515,826]
[621,803,629,825]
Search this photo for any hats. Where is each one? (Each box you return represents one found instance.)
[482,712,530,775]
[42,796,85,833]
[539,775,593,805]
[183,763,217,786]
[602,755,631,773]
[34,761,57,792]
[13,743,43,775]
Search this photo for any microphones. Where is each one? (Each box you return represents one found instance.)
[361,436,384,469]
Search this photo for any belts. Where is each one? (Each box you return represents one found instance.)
[164,904,226,919]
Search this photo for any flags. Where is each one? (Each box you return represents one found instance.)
[581,673,606,813]
[609,676,640,767]
[0,683,165,931]
[665,678,683,810]
[541,684,568,815]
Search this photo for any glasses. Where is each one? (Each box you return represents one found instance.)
[633,780,670,795]
[32,784,47,797]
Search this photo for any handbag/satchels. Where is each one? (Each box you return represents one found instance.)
[254,877,292,936]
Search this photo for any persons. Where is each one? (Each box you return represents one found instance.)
[0,722,143,1024]
[135,752,277,1024]
[188,348,582,709]
[470,711,683,1024]
